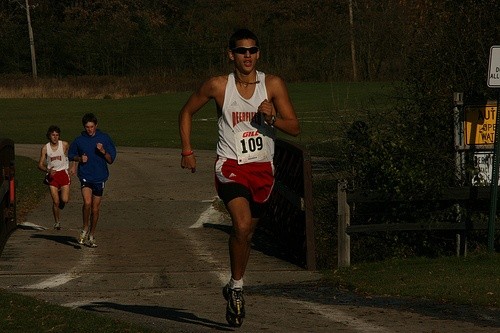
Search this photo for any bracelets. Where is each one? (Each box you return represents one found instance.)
[263,115,276,129]
[183,151,193,155]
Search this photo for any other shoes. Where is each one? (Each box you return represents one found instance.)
[77,227,97,247]
[223,283,245,327]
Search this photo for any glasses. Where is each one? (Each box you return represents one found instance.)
[231,47,258,54]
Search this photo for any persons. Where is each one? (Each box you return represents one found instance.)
[68,113,116,248]
[178,32,299,327]
[38,125,77,227]
